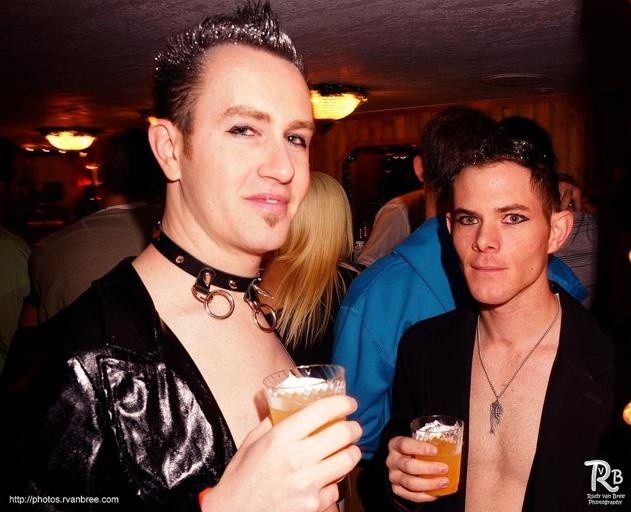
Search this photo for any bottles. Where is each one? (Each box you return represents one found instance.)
[354,226,369,261]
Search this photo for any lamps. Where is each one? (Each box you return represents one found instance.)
[306,87,370,123]
[45,127,100,152]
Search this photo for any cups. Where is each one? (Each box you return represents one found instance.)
[262,364,347,484]
[411,414,465,496]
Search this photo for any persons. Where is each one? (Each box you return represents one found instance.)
[0,0,631,512]
